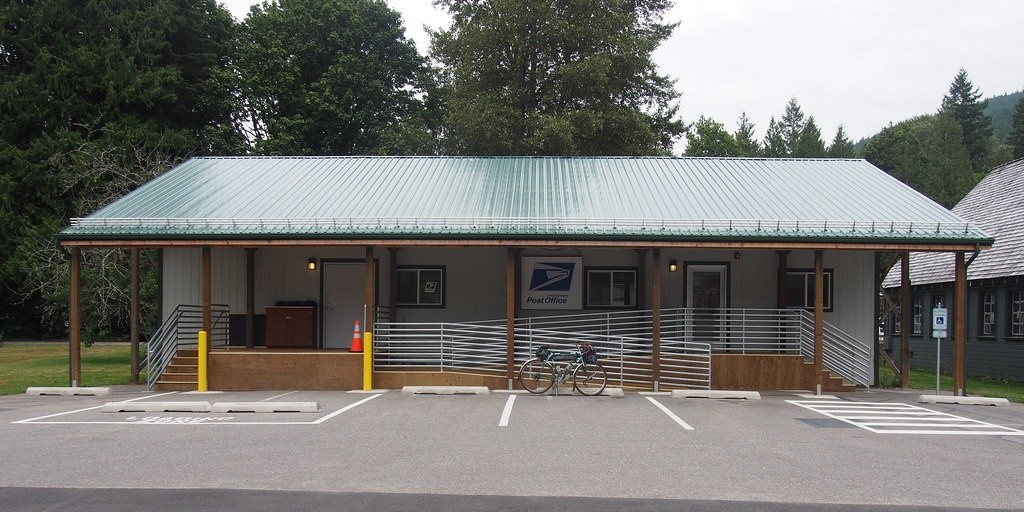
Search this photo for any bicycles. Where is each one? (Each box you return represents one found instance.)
[517,343,607,396]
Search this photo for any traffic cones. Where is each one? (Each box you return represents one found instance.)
[348,320,364,352]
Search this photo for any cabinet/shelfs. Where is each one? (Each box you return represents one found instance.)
[265,305,317,348]
